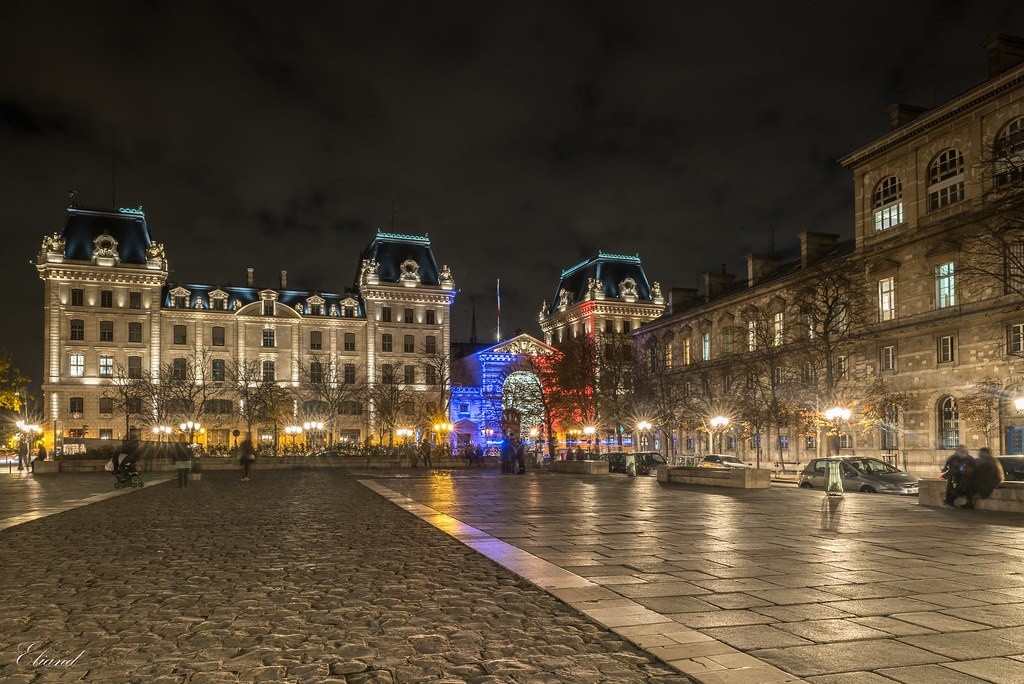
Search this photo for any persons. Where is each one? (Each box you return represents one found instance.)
[465,444,483,467]
[238,431,258,481]
[500,432,526,475]
[566,445,585,460]
[122,425,140,485]
[364,434,375,471]
[173,434,193,488]
[18,438,30,470]
[405,439,433,468]
[30,444,46,473]
[940,443,1004,509]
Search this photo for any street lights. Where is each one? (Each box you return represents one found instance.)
[16,421,30,469]
[825,407,850,455]
[570,430,581,452]
[286,426,302,446]
[304,422,323,448]
[531,429,538,455]
[434,423,454,456]
[181,422,204,443]
[397,429,413,445]
[638,421,651,450]
[481,429,493,455]
[153,427,171,443]
[711,417,729,454]
[584,427,594,452]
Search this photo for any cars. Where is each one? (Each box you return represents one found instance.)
[798,455,923,496]
[595,452,629,473]
[697,454,751,469]
[630,452,669,477]
[972,455,1024,481]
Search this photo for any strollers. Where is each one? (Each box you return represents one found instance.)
[105,450,144,487]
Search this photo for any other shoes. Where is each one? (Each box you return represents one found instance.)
[937,498,955,510]
[516,471,525,474]
[501,472,510,474]
[241,478,249,482]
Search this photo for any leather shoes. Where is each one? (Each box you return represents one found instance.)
[960,502,974,510]
